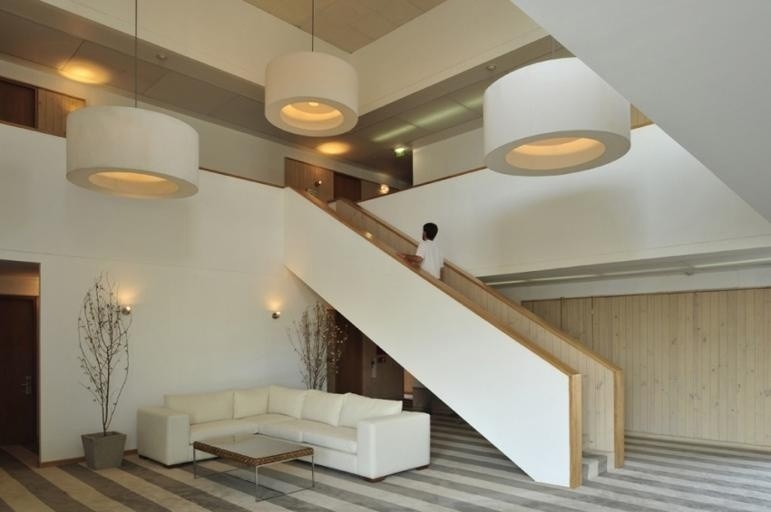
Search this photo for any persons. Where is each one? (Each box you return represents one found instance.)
[398,222,445,284]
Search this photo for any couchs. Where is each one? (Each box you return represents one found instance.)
[137,386,431,483]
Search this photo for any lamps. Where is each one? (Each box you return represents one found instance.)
[66,0,200,200]
[265,0,359,137]
[122,306,131,316]
[314,179,321,187]
[271,311,280,320]
[483,35,632,177]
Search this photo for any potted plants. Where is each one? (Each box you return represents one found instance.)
[75,273,132,469]
